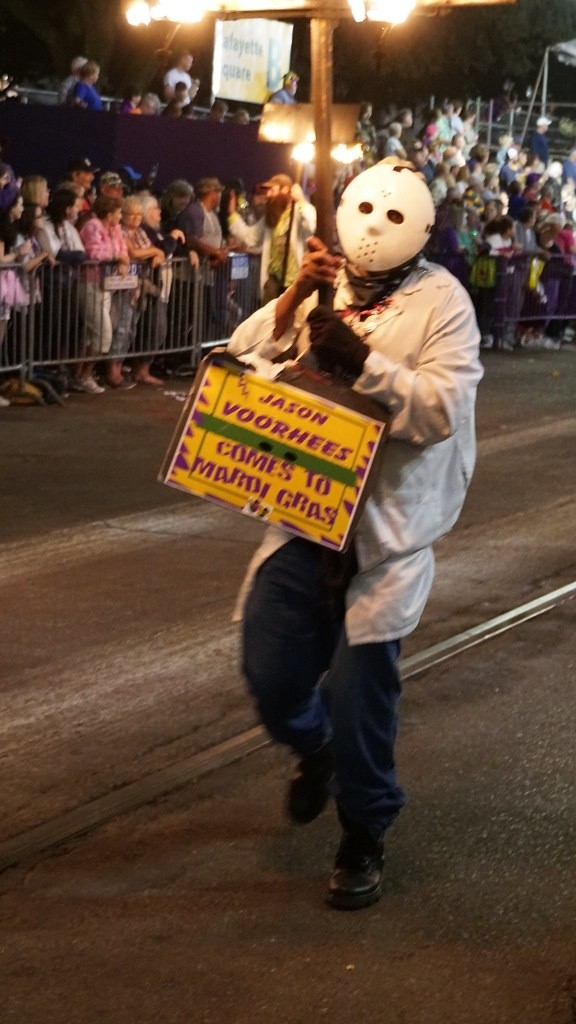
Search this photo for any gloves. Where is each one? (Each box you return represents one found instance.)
[306,306,371,379]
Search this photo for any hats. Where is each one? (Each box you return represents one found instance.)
[260,174,294,188]
[100,172,129,188]
[537,117,553,126]
[70,157,100,173]
[504,147,518,161]
[201,178,226,192]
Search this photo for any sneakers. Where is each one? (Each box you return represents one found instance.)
[72,376,105,392]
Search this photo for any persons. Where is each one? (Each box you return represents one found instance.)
[0,48,576,410]
[231,154,485,911]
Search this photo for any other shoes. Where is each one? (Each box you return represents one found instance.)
[129,371,165,386]
[481,328,573,352]
[106,378,137,390]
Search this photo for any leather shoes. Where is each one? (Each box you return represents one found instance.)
[328,839,386,907]
[286,763,330,821]
